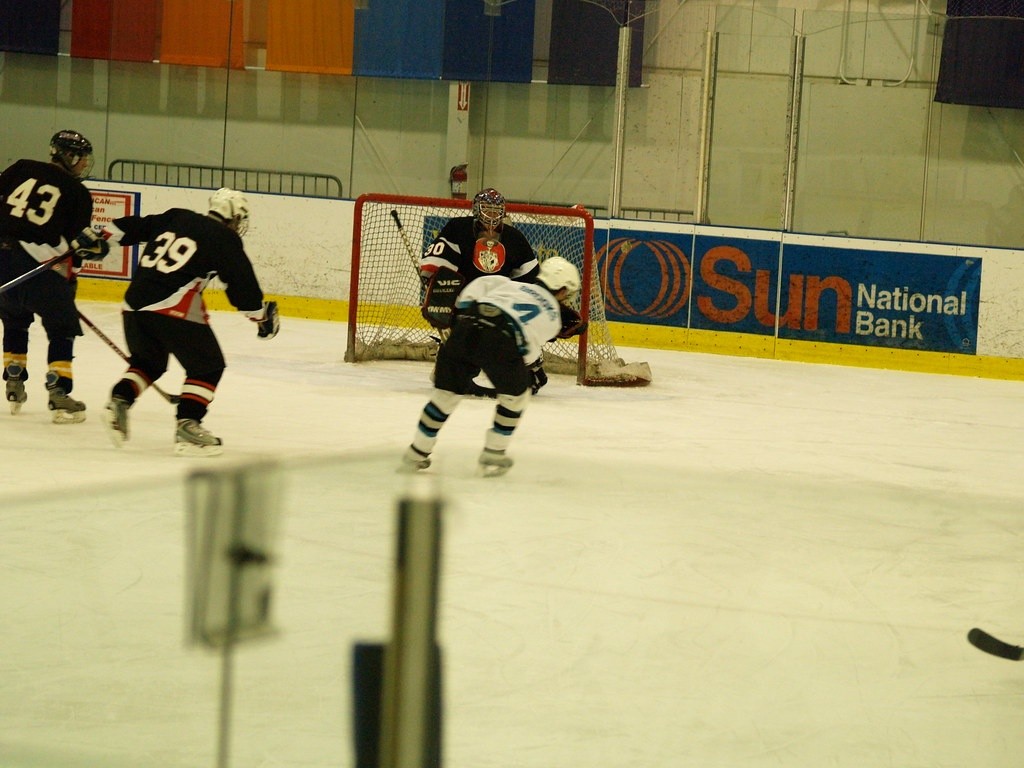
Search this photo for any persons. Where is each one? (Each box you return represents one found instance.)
[0,130,94,425]
[416,185,539,286]
[395,256,582,481]
[70,186,280,456]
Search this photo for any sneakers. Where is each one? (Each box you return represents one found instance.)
[45,372,86,425]
[104,395,129,448]
[174,418,223,456]
[396,455,430,475]
[476,448,513,477]
[5,363,28,415]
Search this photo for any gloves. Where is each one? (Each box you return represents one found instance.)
[252,300,280,341]
[527,359,548,394]
[71,228,110,261]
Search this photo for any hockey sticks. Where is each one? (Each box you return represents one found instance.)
[969,626,1024,665]
[76,306,181,405]
[390,210,500,398]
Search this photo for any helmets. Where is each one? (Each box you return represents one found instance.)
[473,188,506,232]
[536,256,580,294]
[209,187,249,239]
[49,129,95,181]
